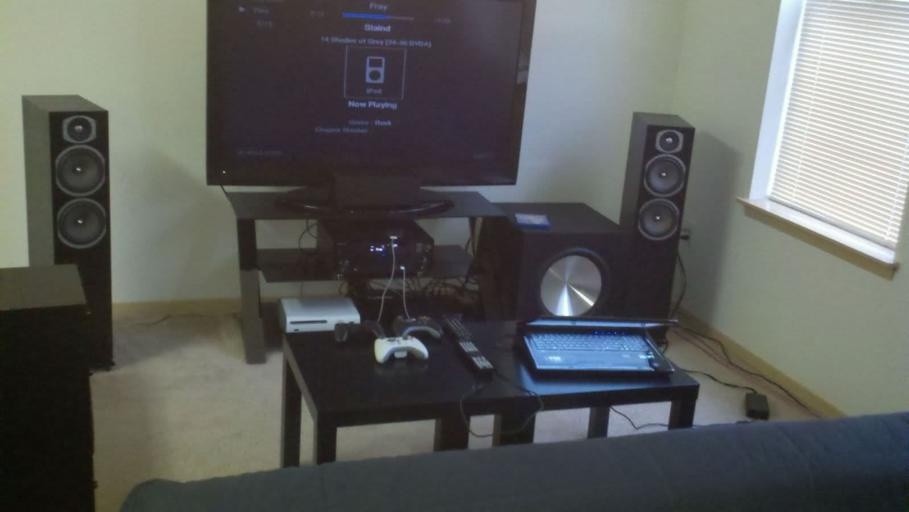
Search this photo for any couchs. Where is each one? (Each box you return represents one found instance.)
[115,385,909,512]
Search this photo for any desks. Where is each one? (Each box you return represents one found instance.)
[279,321,699,466]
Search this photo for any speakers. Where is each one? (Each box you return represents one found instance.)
[484,201,621,316]
[0,264,96,512]
[22,92,116,374]
[616,112,695,344]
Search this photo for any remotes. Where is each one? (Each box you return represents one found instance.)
[459,341,496,373]
[447,317,478,345]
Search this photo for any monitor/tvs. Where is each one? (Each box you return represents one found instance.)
[205,0,538,220]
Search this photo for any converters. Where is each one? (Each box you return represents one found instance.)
[745,393,770,419]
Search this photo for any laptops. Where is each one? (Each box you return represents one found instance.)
[515,319,678,379]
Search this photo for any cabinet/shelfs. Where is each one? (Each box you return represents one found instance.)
[222,189,510,367]
